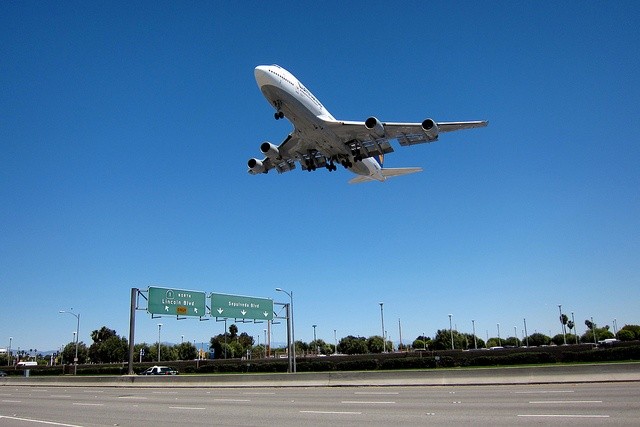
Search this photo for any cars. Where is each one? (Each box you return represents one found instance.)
[540,344,548,346]
[490,347,503,349]
[0,371,7,377]
[519,346,527,348]
[529,346,537,347]
[506,346,516,348]
[140,365,178,375]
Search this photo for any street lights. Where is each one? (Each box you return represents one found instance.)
[275,288,296,371]
[59,310,80,375]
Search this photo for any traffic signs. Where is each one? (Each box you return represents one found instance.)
[211,292,273,320]
[147,286,206,316]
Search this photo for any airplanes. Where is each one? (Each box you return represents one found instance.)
[247,64,489,183]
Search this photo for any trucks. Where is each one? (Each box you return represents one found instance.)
[17,361,38,366]
[598,339,620,346]
